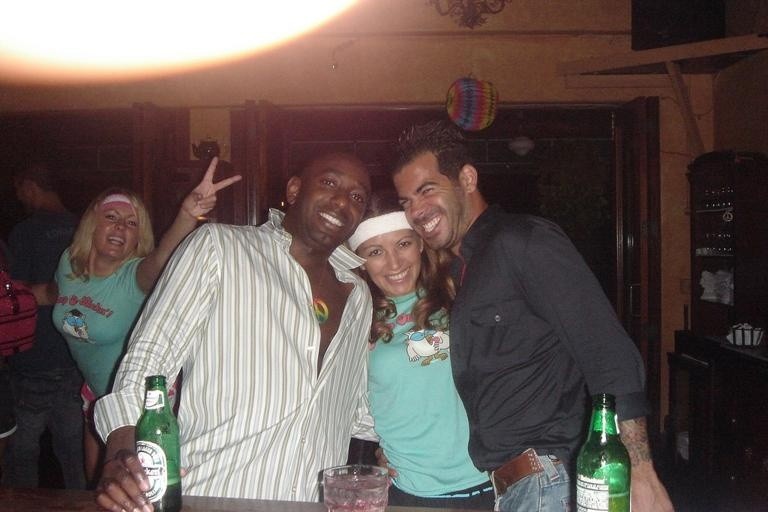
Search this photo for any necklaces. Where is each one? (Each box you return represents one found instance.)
[312,260,329,324]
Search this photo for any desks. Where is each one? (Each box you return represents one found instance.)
[1,484,112,511]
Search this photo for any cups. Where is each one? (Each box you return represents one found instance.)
[323,463,390,511]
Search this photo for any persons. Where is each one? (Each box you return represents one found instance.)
[346,182,495,511]
[7,162,89,491]
[30,155,244,479]
[93,148,379,512]
[374,117,674,512]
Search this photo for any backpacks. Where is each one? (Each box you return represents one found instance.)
[0,267,40,356]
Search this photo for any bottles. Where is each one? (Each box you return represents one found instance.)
[134,375,183,511]
[576,393,632,512]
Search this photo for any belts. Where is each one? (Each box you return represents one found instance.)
[487,447,546,500]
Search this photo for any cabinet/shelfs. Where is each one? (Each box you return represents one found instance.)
[1,102,192,243]
[564,31,768,154]
[155,159,233,244]
[665,150,767,479]
